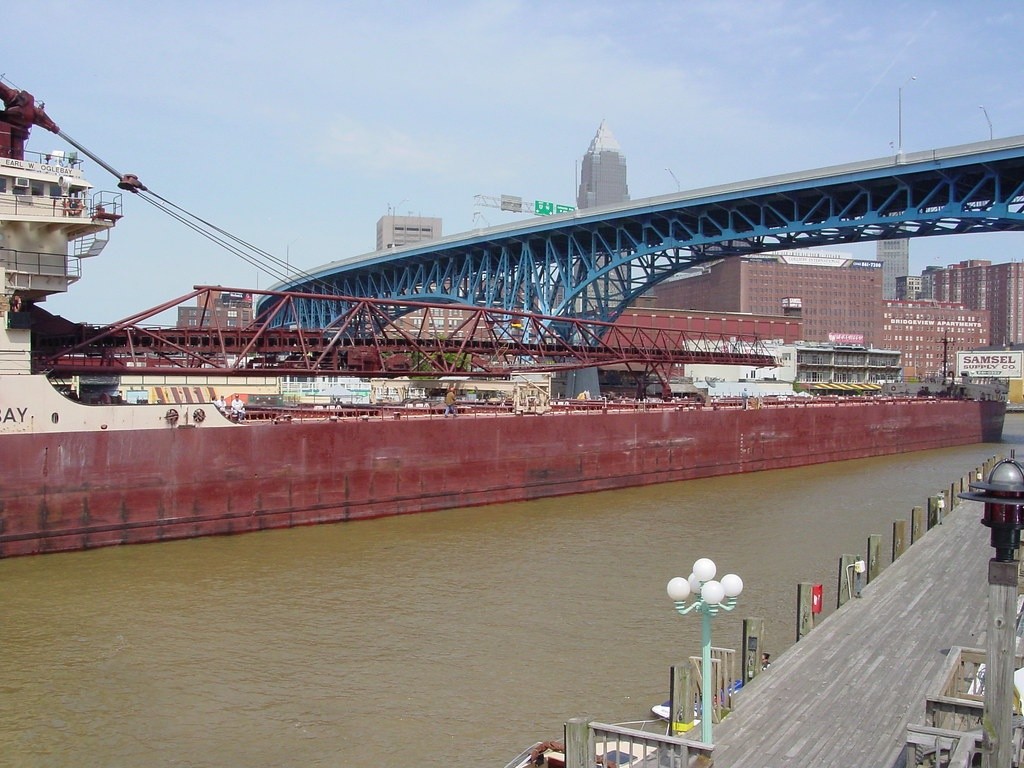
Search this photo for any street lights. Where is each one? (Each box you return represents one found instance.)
[897,76,916,164]
[667,556,744,761]
[664,167,680,192]
[958,447,1024,768]
[979,105,993,140]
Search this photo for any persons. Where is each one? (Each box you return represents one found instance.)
[445,388,459,417]
[742,387,749,408]
[761,654,771,671]
[217,394,244,419]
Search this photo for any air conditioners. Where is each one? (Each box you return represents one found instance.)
[12,178,30,188]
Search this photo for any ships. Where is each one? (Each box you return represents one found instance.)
[0,75,1011,562]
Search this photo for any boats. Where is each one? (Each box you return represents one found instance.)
[651,679,743,724]
[512,738,657,768]
